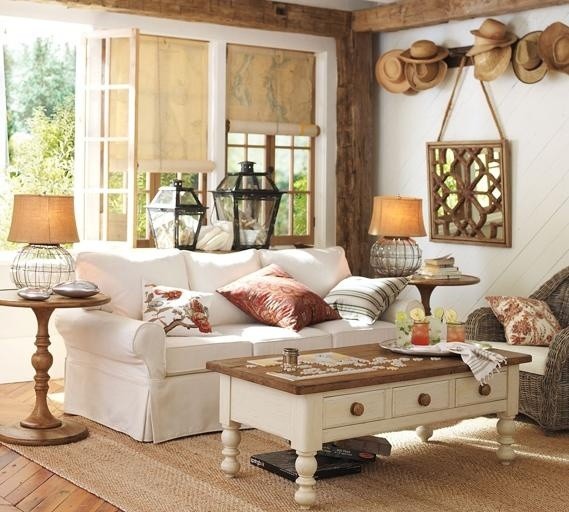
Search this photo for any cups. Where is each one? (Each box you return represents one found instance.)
[395,319,412,347]
[282,347,299,370]
[425,317,441,345]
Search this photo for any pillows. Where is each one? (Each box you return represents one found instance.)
[214,260,343,334]
[135,274,226,338]
[324,273,412,327]
[482,294,563,348]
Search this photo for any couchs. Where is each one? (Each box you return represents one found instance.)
[55,248,427,447]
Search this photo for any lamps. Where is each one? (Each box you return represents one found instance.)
[368,191,429,276]
[212,158,289,252]
[6,192,81,296]
[141,179,210,251]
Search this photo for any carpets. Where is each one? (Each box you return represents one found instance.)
[0,415,569,512]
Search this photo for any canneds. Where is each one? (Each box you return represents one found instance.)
[283,348,298,367]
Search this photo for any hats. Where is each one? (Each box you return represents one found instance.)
[510,29,549,86]
[397,38,452,65]
[537,21,569,77]
[373,48,413,95]
[470,17,514,43]
[472,42,513,83]
[403,60,449,92]
[463,32,519,57]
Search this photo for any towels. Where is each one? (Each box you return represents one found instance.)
[433,339,510,387]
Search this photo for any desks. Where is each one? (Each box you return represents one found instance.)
[405,274,481,317]
[203,342,533,511]
[0,288,112,447]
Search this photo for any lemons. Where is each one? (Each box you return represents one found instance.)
[395,311,406,321]
[446,310,457,322]
[411,308,425,320]
[435,307,443,317]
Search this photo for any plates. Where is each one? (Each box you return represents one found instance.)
[379,338,492,357]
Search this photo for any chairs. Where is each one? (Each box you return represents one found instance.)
[461,265,568,437]
